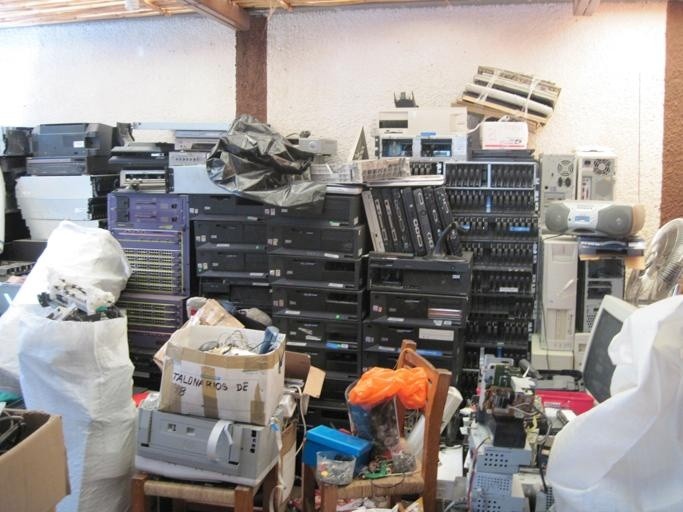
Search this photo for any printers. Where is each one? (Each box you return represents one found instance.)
[26,123,113,176]
[108,138,175,169]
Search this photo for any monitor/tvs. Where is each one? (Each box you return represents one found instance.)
[580,294,638,406]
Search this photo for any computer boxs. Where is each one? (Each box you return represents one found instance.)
[577,151,616,201]
[583,255,625,332]
[537,235,579,351]
[538,154,577,233]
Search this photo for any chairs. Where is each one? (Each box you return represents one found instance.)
[299,340,452,511]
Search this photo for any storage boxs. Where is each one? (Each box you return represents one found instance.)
[280,351,327,415]
[0,407,72,512]
[279,417,298,511]
[157,323,287,425]
[301,424,373,480]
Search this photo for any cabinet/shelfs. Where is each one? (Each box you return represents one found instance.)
[132,453,282,511]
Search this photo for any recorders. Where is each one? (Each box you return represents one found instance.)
[546,201,645,238]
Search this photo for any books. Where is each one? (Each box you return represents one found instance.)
[451,66,562,134]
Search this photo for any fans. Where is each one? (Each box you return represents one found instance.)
[625,216,683,311]
